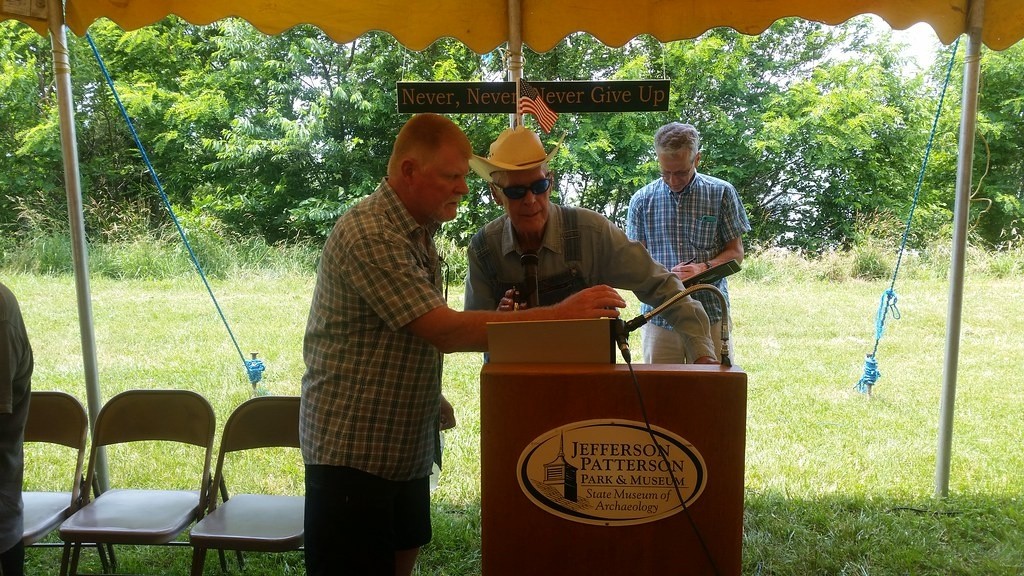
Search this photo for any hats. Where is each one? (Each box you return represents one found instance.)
[469,125,568,181]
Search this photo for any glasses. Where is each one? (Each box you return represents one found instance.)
[658,156,695,177]
[490,179,551,200]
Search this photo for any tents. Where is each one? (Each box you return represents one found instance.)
[0,1,1023,495]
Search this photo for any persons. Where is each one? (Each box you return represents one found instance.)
[627,123,751,363]
[299,114,626,576]
[464,126,721,365]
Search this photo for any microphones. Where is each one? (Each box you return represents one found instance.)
[520,250,538,308]
[604,306,631,363]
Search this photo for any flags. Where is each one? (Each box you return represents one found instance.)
[519,78,559,134]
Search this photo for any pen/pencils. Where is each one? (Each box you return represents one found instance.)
[684,256,698,266]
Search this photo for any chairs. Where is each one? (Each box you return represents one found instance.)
[19,389,110,576]
[189,395,306,576]
[58,389,228,576]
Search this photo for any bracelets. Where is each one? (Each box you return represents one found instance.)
[703,260,712,269]
